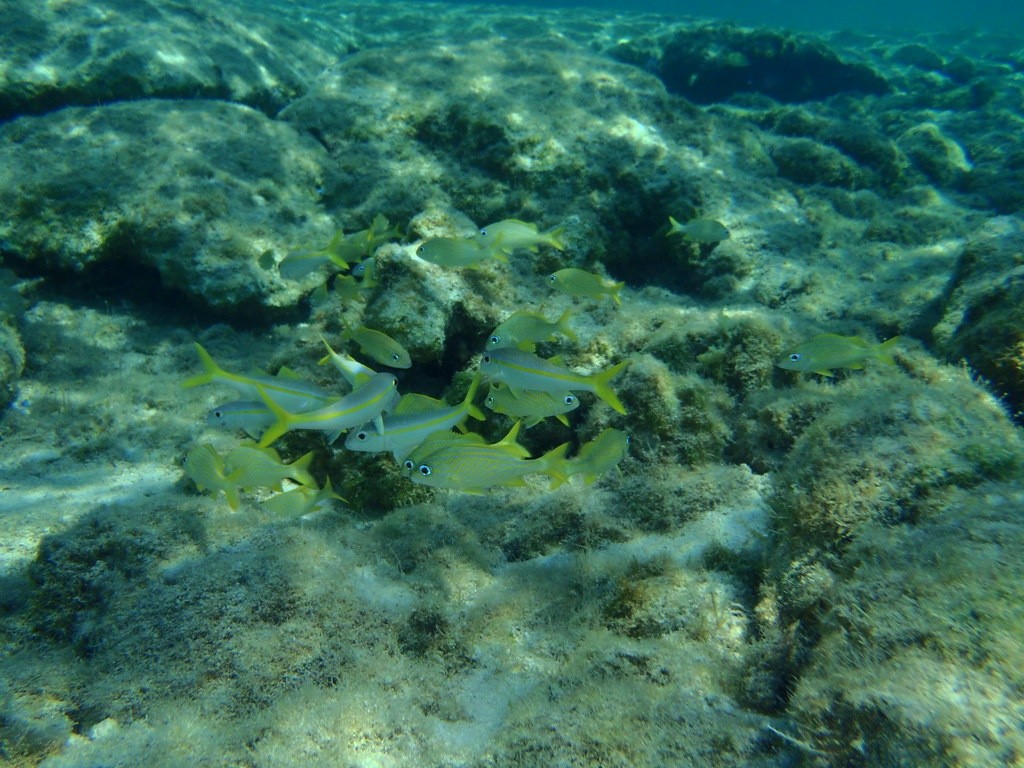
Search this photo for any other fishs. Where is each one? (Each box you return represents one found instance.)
[170,208,633,527]
[665,215,729,242]
[777,334,903,378]
